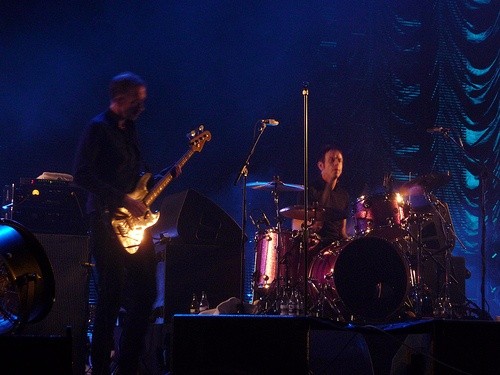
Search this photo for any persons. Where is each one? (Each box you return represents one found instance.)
[292,146,353,240]
[78,73,182,375]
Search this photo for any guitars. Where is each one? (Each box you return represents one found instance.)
[107,125,213,255]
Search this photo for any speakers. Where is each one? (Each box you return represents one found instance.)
[389,333,450,375]
[152,189,250,312]
[17,233,91,375]
[307,328,374,375]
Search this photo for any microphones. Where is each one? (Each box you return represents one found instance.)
[261,119,279,126]
[261,212,270,226]
[434,127,449,132]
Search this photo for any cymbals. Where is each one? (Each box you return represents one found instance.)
[279,204,328,221]
[399,175,449,195]
[246,180,307,192]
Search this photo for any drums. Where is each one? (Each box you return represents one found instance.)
[252,228,305,294]
[308,234,411,323]
[350,193,410,241]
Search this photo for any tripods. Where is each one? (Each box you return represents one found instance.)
[252,185,346,324]
